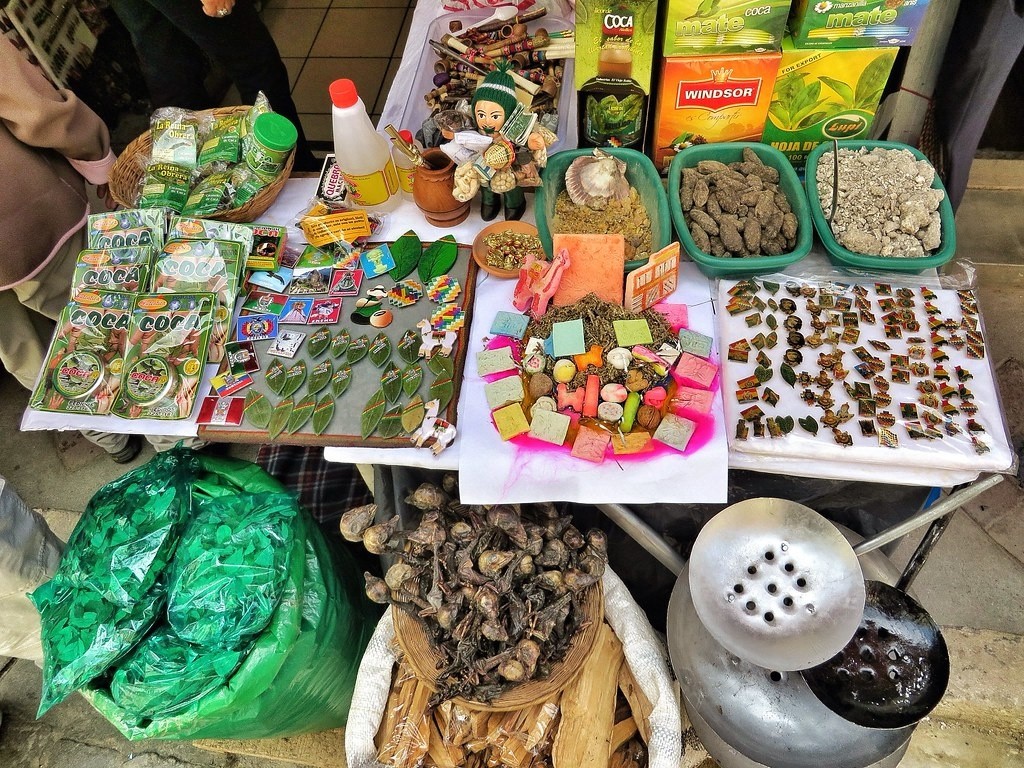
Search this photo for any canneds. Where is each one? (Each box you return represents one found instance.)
[246,113,298,176]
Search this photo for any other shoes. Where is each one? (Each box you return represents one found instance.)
[109,434,146,465]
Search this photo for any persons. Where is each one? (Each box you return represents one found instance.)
[0,32,211,670]
[442,59,543,222]
[109,0,330,172]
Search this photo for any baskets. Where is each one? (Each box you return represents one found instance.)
[109,105,296,224]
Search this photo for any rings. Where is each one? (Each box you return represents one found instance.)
[217,9,228,16]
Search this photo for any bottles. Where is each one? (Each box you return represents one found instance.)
[392,130,422,200]
[330,79,401,215]
[578,5,645,148]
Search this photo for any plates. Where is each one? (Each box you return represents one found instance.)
[473,220,546,278]
